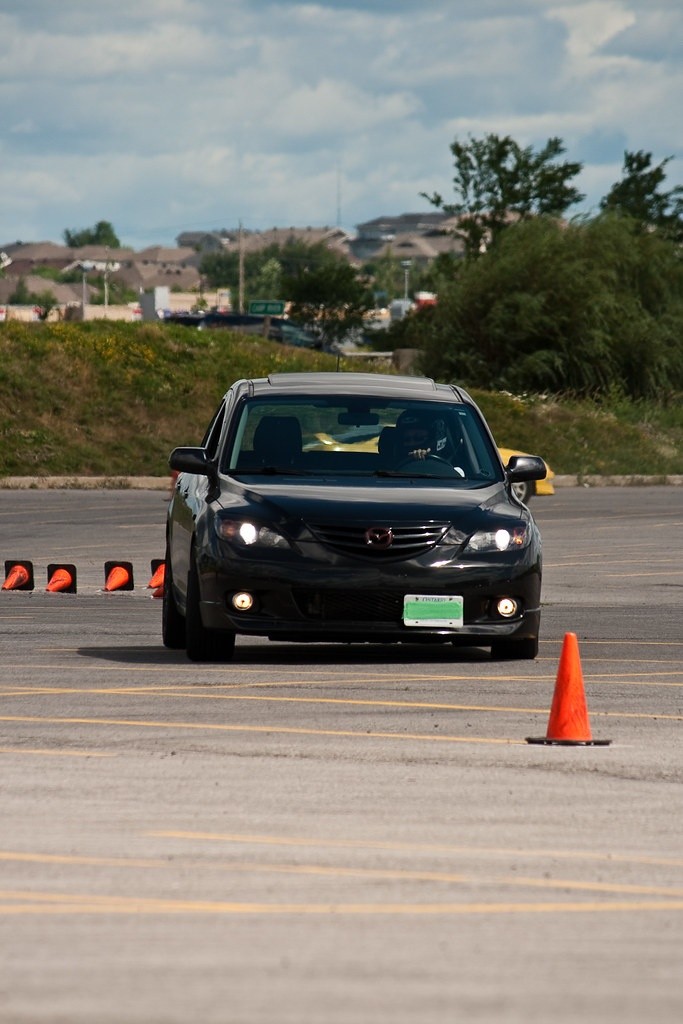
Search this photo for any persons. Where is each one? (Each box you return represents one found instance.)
[379,409,466,478]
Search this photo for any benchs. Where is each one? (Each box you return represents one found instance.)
[229,450,378,470]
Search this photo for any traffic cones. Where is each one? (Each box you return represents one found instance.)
[148,559,166,588]
[2,560,34,591]
[152,585,164,598]
[526,633,611,746]
[46,564,76,594]
[104,561,134,592]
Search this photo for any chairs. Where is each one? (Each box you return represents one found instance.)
[378,426,397,473]
[253,415,303,474]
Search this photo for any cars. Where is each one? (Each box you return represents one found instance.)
[162,371,547,661]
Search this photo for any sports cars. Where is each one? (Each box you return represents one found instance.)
[301,421,555,506]
